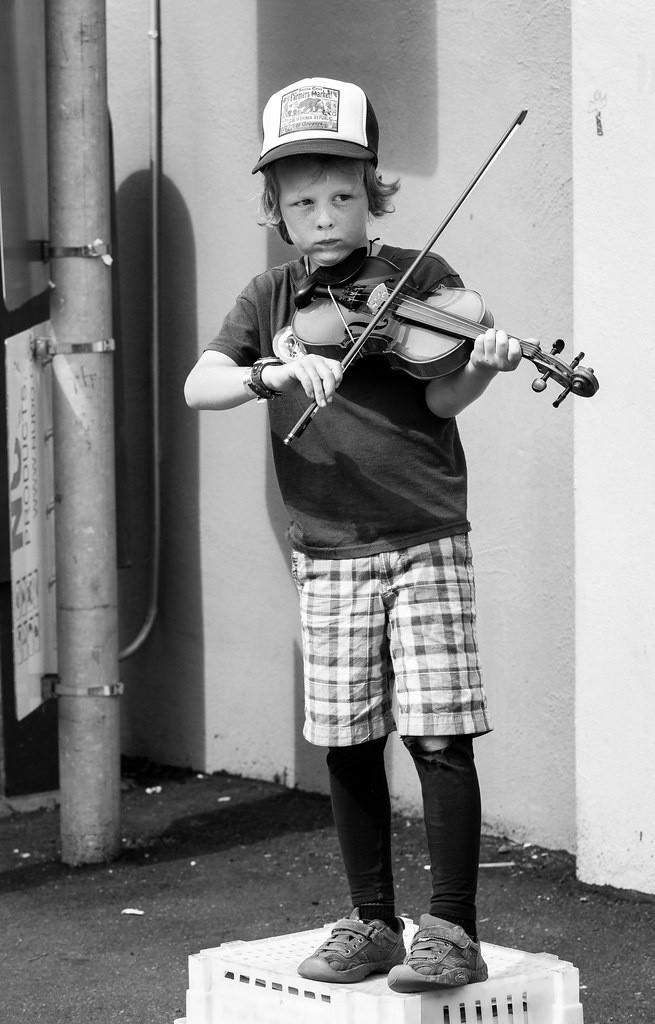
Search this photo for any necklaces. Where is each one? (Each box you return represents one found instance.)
[299,234,380,282]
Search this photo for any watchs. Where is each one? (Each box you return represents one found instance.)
[247,353,288,401]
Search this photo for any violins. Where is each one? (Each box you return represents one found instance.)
[291,248,600,410]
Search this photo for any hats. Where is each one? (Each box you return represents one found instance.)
[252,77,379,172]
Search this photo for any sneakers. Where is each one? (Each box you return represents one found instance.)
[298,906,408,983]
[387,914,489,994]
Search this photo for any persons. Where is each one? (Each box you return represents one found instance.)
[178,73,549,995]
